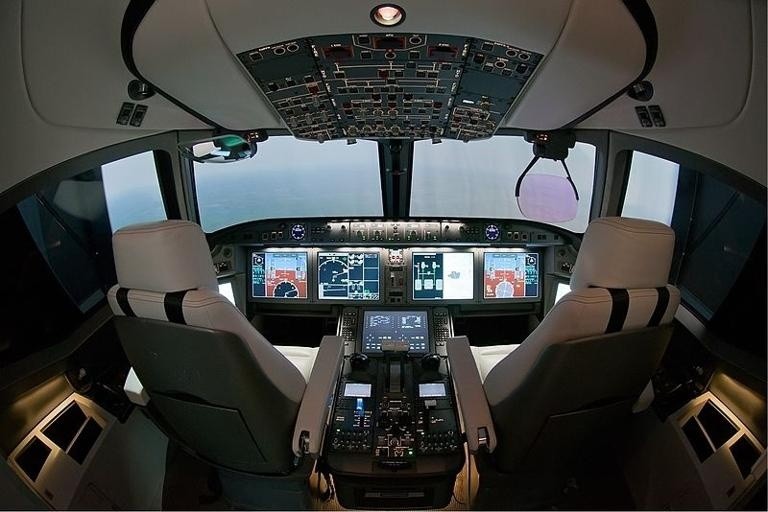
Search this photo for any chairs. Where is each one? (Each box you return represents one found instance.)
[104,220,343,510]
[450,216,683,508]
